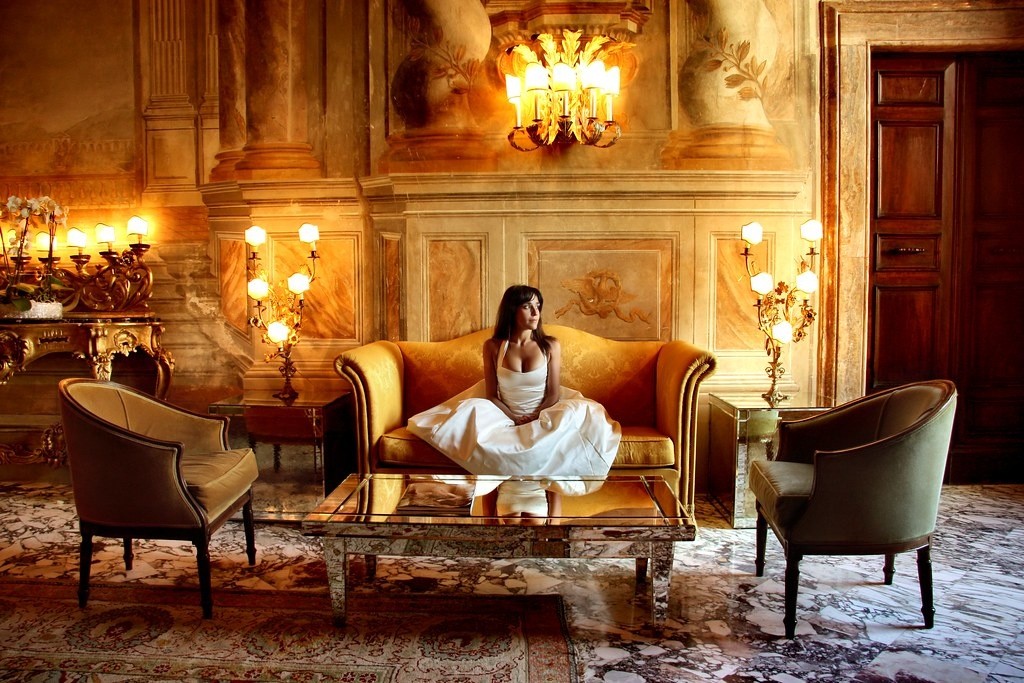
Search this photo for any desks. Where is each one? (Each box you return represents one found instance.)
[0,321,175,482]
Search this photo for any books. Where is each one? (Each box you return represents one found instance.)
[396,483,476,512]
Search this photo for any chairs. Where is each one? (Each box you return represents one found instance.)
[750,380,958,640]
[59,377,257,621]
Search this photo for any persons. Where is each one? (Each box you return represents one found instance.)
[483,286,561,475]
[482,475,562,525]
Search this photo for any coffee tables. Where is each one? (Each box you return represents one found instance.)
[299,473,696,636]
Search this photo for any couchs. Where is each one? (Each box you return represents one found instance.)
[332,323,718,522]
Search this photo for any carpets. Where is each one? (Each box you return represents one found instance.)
[0,576,578,683]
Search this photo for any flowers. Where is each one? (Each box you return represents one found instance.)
[0,194,68,301]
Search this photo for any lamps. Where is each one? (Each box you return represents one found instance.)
[504,57,622,153]
[245,222,321,397]
[740,218,826,404]
[0,215,154,312]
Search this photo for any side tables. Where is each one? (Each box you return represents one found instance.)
[709,392,842,530]
[207,389,352,523]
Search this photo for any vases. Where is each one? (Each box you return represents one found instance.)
[0,300,63,322]
